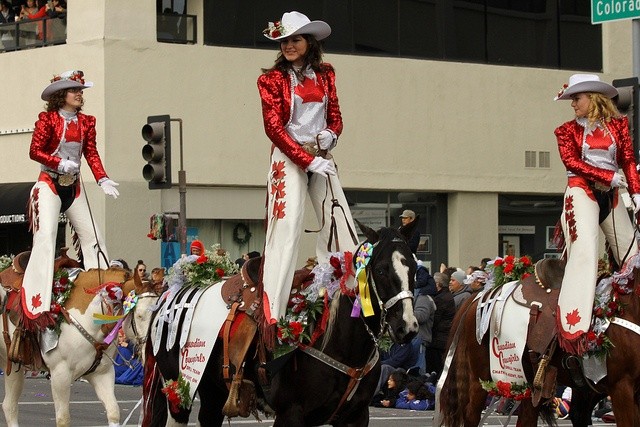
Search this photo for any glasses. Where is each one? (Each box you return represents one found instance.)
[68,88,84,94]
[138,267,147,273]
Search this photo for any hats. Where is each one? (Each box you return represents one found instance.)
[398,209,416,219]
[263,10,332,42]
[463,269,486,285]
[451,271,467,286]
[553,73,619,102]
[41,70,95,101]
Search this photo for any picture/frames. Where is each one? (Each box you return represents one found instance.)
[416,233,431,254]
[546,224,565,249]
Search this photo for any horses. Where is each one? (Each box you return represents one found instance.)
[140,216,424,427]
[1,261,161,426]
[435,255,640,426]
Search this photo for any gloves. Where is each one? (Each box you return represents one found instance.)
[62,160,80,176]
[610,171,628,189]
[314,129,334,151]
[305,156,337,178]
[632,192,640,214]
[101,180,120,199]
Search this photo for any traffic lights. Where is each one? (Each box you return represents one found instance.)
[611,76,640,168]
[141,114,172,189]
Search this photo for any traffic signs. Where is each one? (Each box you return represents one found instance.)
[591,0,640,25]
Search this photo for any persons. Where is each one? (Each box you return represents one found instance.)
[396,379,436,410]
[136,259,147,277]
[190,239,207,264]
[449,271,471,313]
[117,328,134,354]
[413,258,438,303]
[149,267,159,284]
[463,270,487,290]
[398,208,422,294]
[430,272,457,369]
[443,267,457,281]
[13,69,120,336]
[467,266,479,274]
[109,258,132,273]
[377,336,420,394]
[479,257,492,270]
[412,294,438,374]
[374,370,437,408]
[256,9,361,344]
[552,72,639,355]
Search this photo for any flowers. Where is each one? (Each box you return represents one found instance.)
[487,255,535,286]
[289,292,325,320]
[159,374,194,415]
[186,242,237,287]
[586,300,624,350]
[277,315,326,345]
[48,268,74,316]
[147,233,158,240]
[481,378,533,413]
